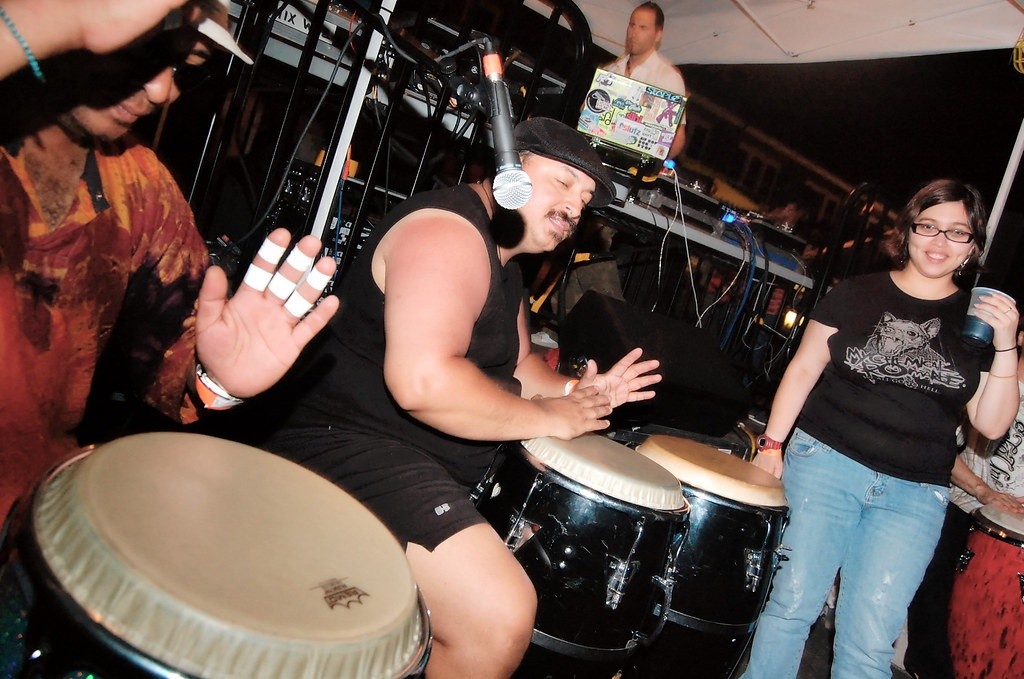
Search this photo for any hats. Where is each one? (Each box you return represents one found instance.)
[514,118,616,210]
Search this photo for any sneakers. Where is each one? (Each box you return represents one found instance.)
[531,332,558,350]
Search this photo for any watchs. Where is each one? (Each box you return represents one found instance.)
[755,434,783,452]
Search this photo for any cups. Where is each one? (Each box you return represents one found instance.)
[959,287,1017,346]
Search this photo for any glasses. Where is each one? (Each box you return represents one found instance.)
[911,221,974,245]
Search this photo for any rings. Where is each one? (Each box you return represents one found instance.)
[1003,307,1012,314]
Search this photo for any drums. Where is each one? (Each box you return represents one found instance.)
[0,429,434,679]
[481,431,692,679]
[944,500,1024,679]
[632,435,790,679]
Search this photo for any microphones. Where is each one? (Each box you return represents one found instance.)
[483,38,532,209]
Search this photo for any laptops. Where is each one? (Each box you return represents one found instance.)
[577,68,688,161]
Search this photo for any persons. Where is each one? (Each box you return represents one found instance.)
[0,0,341,679]
[736,178,1024,679]
[251,114,663,679]
[576,0,688,260]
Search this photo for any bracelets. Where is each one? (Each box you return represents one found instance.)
[0,5,48,80]
[194,363,246,409]
[994,345,1018,352]
[989,371,1018,379]
[564,380,580,396]
